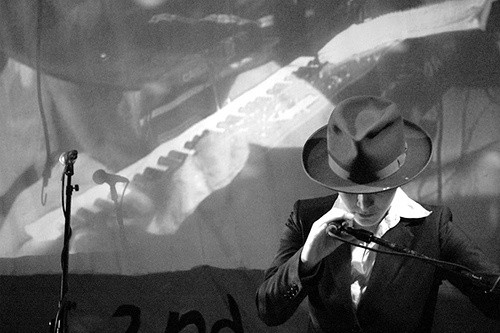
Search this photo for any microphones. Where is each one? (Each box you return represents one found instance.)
[59,150,78,165]
[327,217,354,234]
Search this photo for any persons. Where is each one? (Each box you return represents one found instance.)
[1,1,341,272]
[254,95,500,333]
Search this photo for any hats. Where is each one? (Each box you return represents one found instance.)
[302,95,434,193]
